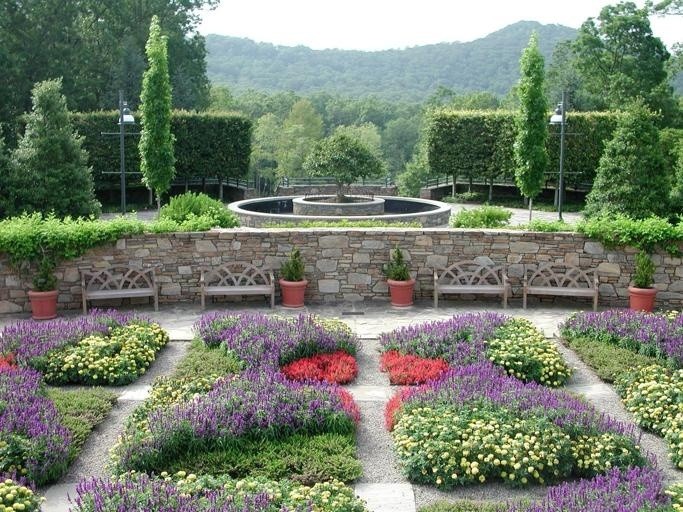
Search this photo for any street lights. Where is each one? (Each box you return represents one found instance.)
[550,88,568,221]
[118,90,132,218]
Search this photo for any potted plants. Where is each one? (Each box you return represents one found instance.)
[25,255,61,321]
[382,246,418,308]
[278,248,310,308]
[627,249,664,312]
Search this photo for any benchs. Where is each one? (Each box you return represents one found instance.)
[198,259,275,311]
[432,259,510,309]
[77,265,160,314]
[523,260,600,312]
